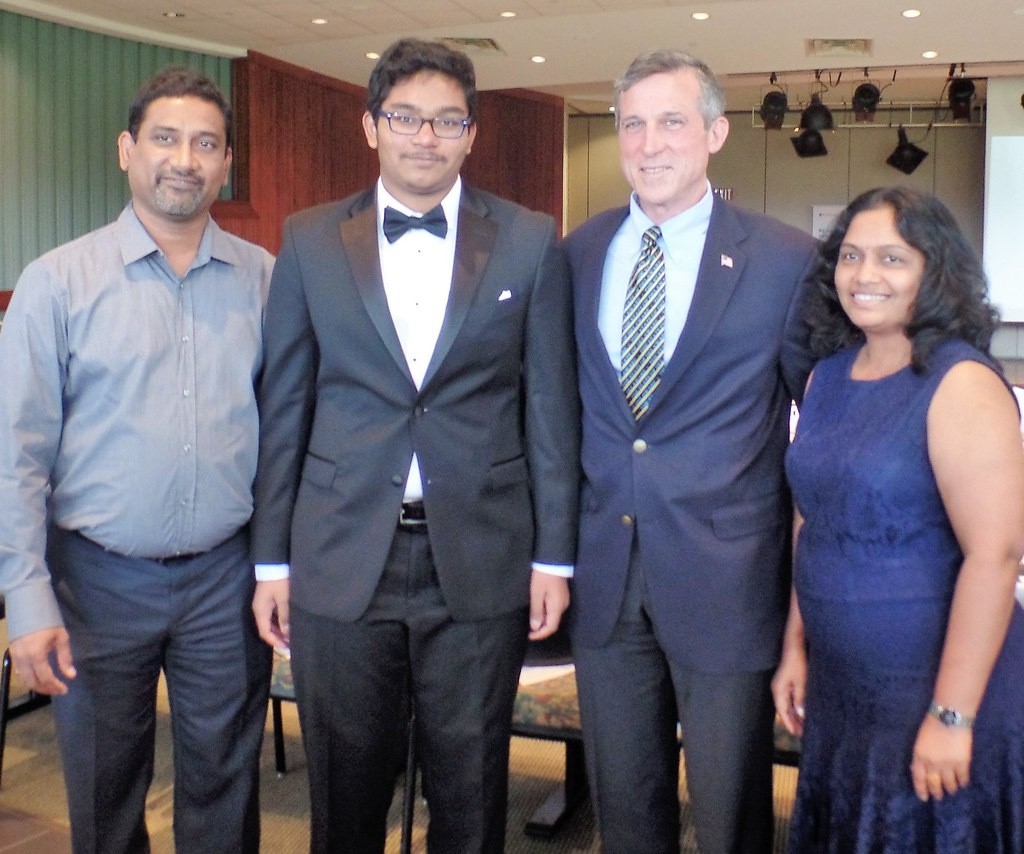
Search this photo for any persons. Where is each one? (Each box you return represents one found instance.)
[244,37,583,854]
[1,70,281,853]
[564,49,827,853]
[769,186,1024,853]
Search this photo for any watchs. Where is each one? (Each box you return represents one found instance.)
[929,703,975,729]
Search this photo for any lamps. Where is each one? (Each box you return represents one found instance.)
[760,63,977,176]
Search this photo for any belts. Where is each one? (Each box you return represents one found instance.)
[396,501,428,534]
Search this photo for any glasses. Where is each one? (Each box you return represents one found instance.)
[377,109,472,139]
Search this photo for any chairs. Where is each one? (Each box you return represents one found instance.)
[268,639,800,854]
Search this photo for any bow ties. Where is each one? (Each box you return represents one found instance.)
[383,203,447,243]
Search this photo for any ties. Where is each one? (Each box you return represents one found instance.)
[621,227,665,424]
